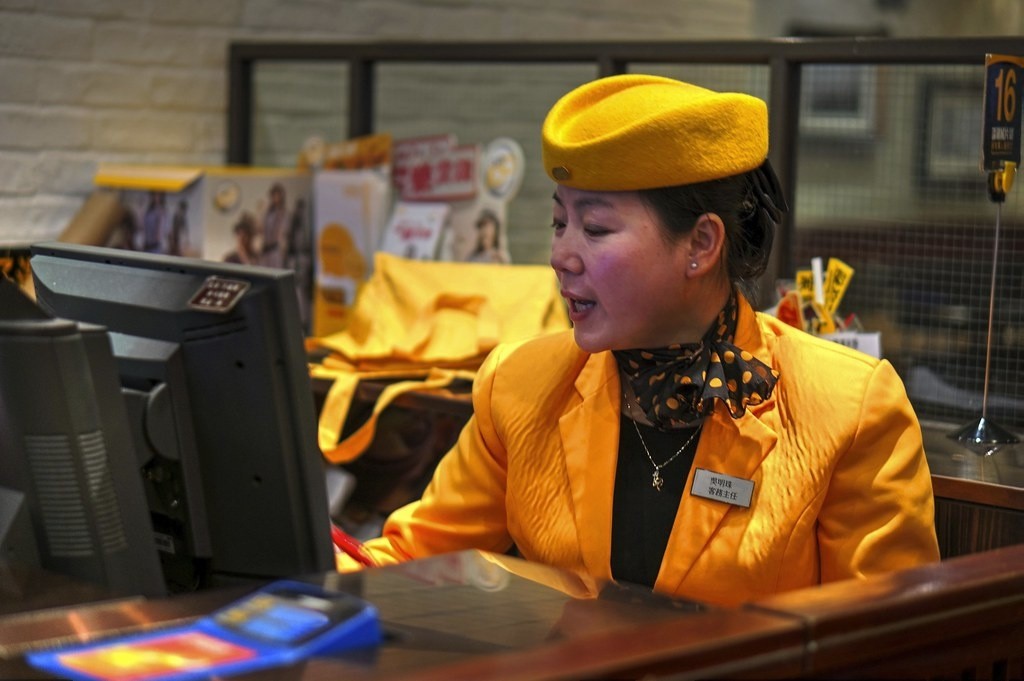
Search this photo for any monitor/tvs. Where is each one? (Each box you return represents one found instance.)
[0,241,336,615]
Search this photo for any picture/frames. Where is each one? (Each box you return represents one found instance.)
[787,19,886,144]
[918,74,994,195]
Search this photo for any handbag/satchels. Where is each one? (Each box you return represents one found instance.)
[307,250,568,463]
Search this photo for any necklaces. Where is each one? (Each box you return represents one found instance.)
[629,402,703,491]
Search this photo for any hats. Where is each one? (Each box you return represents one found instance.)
[542,73,769,191]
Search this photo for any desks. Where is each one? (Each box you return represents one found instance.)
[0,545,704,681]
[304,375,1024,560]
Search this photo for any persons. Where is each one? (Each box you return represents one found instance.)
[143,189,167,257]
[465,209,504,264]
[336,73,943,611]
[167,197,190,256]
[224,183,310,327]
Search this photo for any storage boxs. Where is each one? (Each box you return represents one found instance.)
[94,161,316,334]
